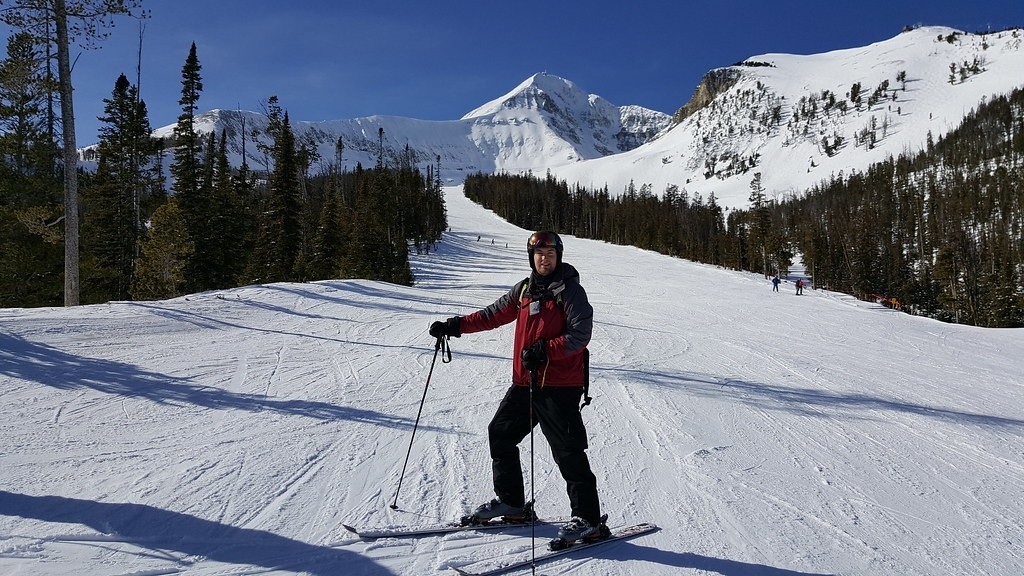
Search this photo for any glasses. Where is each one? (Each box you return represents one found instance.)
[529,233,558,247]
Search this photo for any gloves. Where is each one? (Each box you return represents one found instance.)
[429,316,463,339]
[523,338,547,371]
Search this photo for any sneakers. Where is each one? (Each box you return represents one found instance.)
[476,500,524,518]
[558,515,599,541]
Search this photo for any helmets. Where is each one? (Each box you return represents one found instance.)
[527,231,563,272]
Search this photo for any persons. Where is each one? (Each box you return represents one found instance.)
[772,275,780,292]
[795,278,804,295]
[431,231,613,545]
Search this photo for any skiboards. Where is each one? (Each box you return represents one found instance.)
[343,515,657,576]
